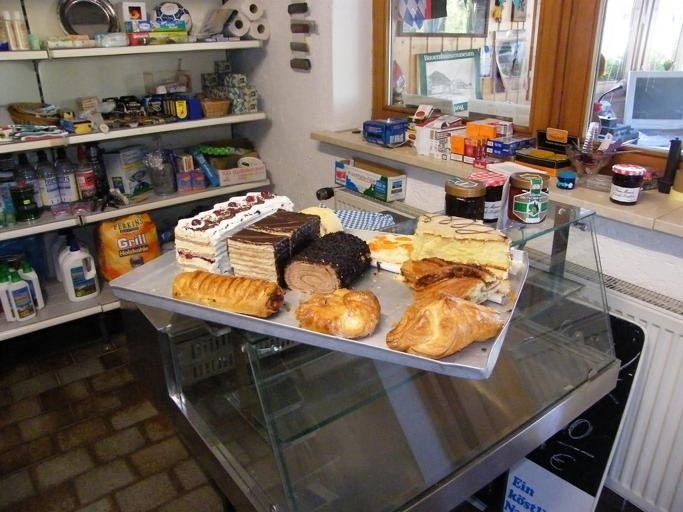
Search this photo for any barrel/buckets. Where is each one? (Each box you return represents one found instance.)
[52,228,101,303]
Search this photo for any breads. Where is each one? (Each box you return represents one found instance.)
[170,190,512,360]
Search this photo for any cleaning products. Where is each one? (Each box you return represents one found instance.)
[0,261,45,321]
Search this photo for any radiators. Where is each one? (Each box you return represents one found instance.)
[334,185,683,512]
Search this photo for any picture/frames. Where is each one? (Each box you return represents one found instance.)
[416,49,480,100]
[397,0,491,37]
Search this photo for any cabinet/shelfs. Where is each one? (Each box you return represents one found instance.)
[1,40,270,341]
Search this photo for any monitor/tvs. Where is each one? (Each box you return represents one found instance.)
[620,69,683,135]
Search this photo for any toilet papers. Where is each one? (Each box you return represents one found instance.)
[218,0,271,41]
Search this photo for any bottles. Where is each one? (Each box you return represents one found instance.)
[0,255,46,324]
[1,142,110,227]
[1,9,31,51]
[555,170,577,190]
